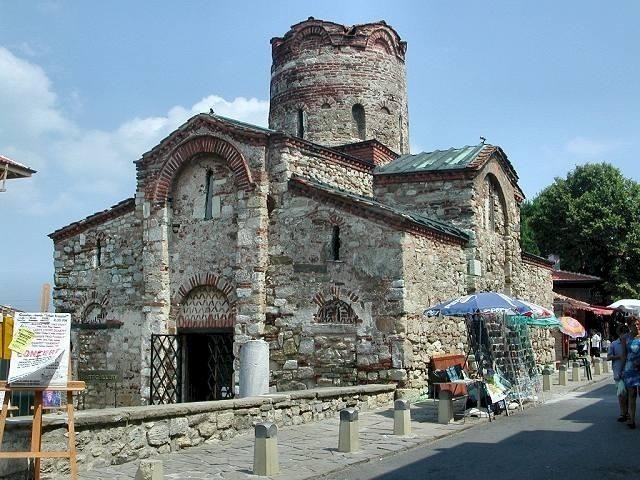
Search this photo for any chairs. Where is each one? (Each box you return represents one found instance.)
[571,355,592,384]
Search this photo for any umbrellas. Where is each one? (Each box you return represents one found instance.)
[506,300,559,330]
[556,316,586,361]
[607,297,640,318]
[422,292,533,418]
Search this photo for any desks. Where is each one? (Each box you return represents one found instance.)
[432,373,491,423]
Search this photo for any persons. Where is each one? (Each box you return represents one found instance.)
[621,316,639,428]
[577,329,603,355]
[606,325,631,423]
